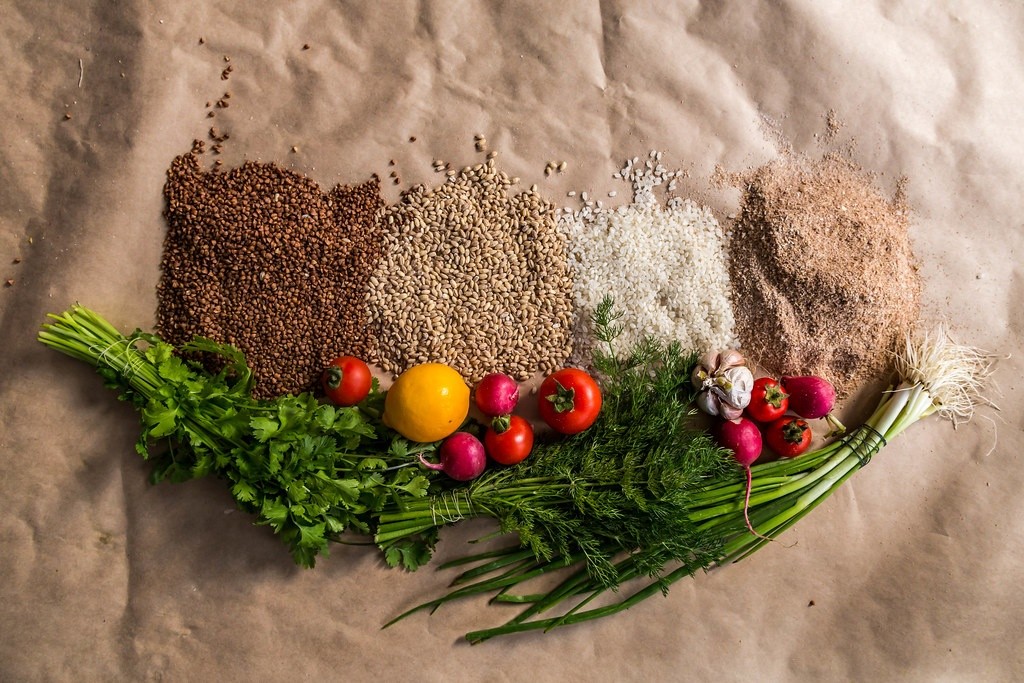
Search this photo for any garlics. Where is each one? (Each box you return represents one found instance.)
[691,350,753,419]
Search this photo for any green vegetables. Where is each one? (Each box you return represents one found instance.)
[370,293,745,600]
[34,298,486,575]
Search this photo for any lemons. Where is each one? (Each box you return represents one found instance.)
[381,363,470,443]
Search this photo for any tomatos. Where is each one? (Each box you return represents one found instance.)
[322,356,372,406]
[537,367,602,435]
[484,414,534,465]
[745,378,788,422]
[765,415,812,457]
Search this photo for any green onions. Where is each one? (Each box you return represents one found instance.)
[376,334,1011,642]
